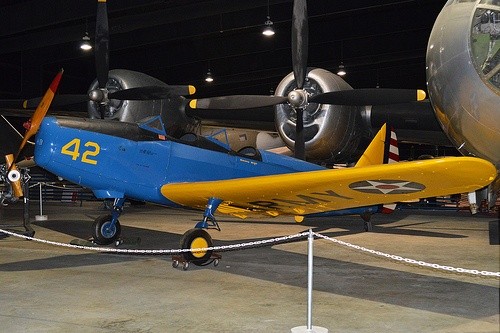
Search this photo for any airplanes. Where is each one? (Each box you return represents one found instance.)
[0,0,500,274]
